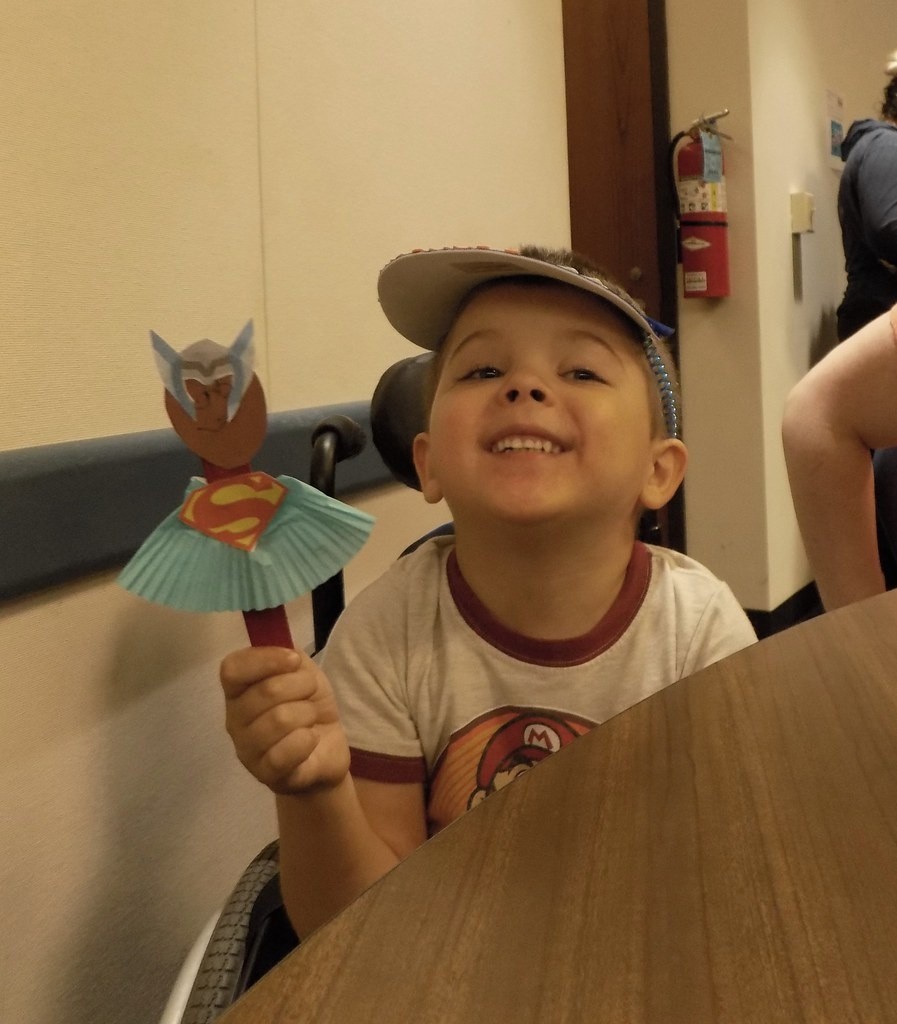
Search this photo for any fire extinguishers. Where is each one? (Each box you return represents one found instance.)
[670,106,735,301]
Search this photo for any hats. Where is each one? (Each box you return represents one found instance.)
[378,249,678,437]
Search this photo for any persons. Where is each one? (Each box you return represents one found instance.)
[836,73,897,593]
[782,302,897,610]
[220,243,761,941]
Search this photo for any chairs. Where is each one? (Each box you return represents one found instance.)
[174,352,454,1024]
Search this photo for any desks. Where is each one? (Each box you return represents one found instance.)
[210,587,897,1024]
